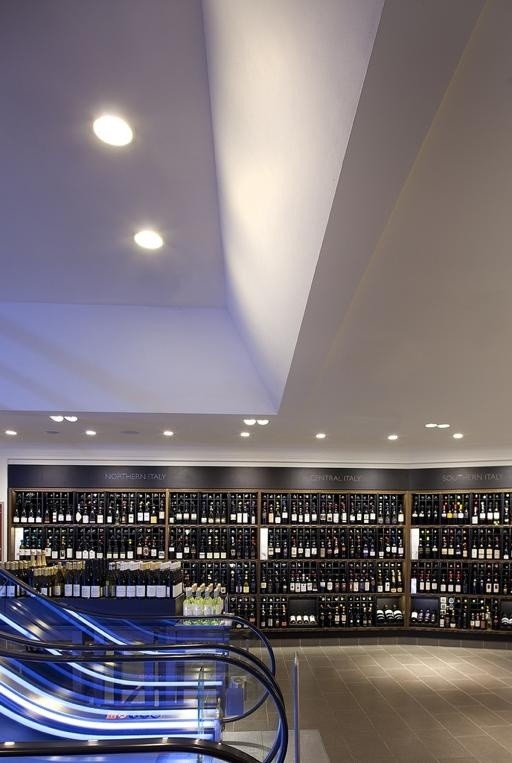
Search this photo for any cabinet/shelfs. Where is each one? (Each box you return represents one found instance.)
[5,486,512,637]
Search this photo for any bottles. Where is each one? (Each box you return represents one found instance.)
[0,492,510,628]
